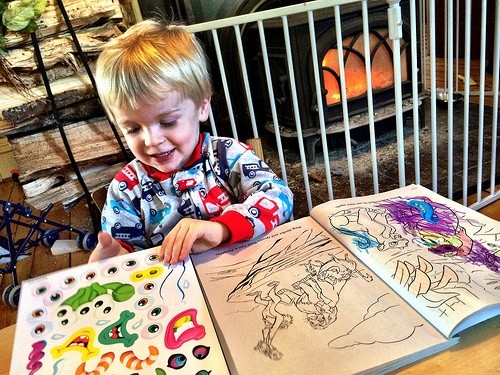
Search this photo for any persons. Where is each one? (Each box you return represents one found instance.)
[87,18,294,265]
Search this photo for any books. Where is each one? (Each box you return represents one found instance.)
[189,183,500,375]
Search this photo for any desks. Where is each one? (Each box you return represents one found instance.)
[0,315,499,374]
